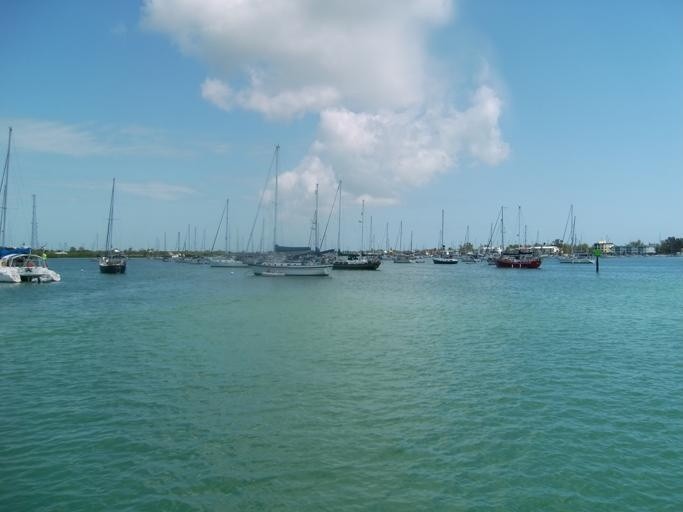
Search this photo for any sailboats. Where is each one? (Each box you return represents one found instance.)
[97,177,127,275]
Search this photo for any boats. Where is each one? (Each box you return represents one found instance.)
[0,252,61,283]
